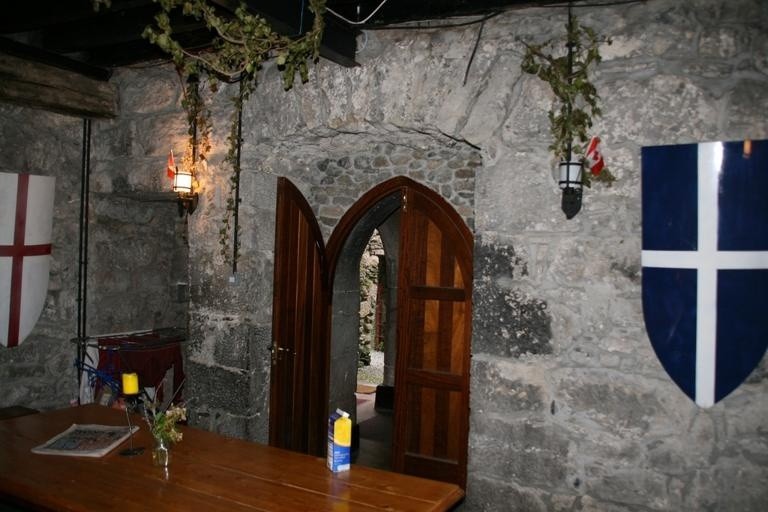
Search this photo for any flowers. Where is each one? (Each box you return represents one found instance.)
[139,364,193,445]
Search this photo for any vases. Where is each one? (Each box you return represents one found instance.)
[150,439,171,468]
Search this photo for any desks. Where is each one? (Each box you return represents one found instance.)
[96,333,187,402]
[0,403,465,510]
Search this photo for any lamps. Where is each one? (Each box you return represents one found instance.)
[172,171,200,215]
[558,156,583,221]
